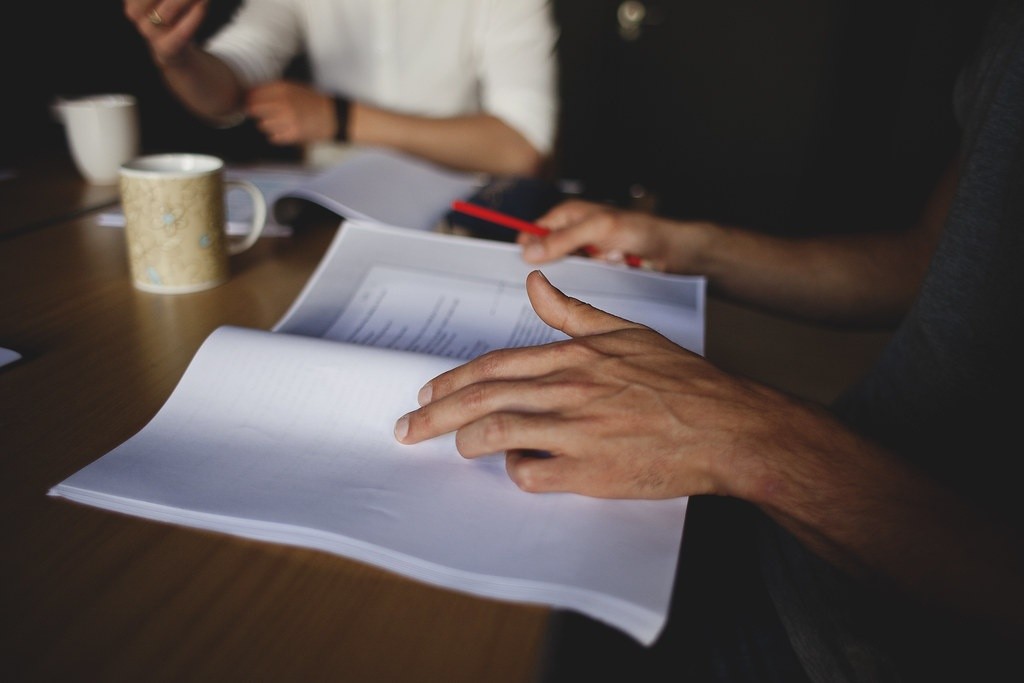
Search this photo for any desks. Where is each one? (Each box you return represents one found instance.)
[0,202,561,683]
[0,147,115,241]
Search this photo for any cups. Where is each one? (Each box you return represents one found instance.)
[120,154,267,294]
[60,95,140,186]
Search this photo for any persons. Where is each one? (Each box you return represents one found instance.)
[393,0,1024,683]
[122,0,561,237]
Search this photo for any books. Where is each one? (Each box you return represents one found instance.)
[91,147,492,239]
[42,215,707,647]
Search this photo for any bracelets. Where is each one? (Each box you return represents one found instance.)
[330,90,354,143]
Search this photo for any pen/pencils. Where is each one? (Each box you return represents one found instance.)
[452,200,656,271]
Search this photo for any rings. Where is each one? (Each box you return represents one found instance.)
[147,7,169,27]
